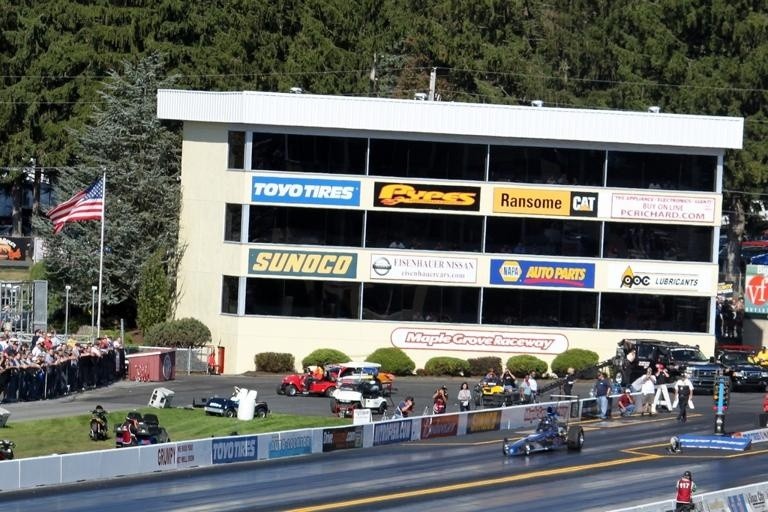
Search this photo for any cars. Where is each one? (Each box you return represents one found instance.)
[718,223,767,274]
[0,280,32,329]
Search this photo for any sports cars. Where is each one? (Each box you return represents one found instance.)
[501,409,584,457]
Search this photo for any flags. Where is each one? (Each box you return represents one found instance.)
[46,173,103,234]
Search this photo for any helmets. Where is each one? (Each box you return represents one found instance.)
[683,470,693,480]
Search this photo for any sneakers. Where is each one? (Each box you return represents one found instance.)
[594,415,608,420]
[676,418,688,424]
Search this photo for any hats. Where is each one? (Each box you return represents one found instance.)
[440,383,449,391]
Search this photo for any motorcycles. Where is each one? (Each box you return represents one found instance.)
[0,440,15,460]
[88,405,110,441]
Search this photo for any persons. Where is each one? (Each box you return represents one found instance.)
[0,321,121,403]
[457,369,538,412]
[432,384,448,415]
[391,396,415,419]
[563,367,578,399]
[304,362,325,392]
[675,471,698,512]
[757,345,768,366]
[716,295,745,339]
[370,374,382,391]
[592,363,694,424]
[747,349,759,364]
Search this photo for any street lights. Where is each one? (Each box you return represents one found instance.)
[92,286,97,338]
[65,285,71,344]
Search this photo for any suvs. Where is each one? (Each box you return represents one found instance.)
[616,338,717,396]
[715,349,767,391]
